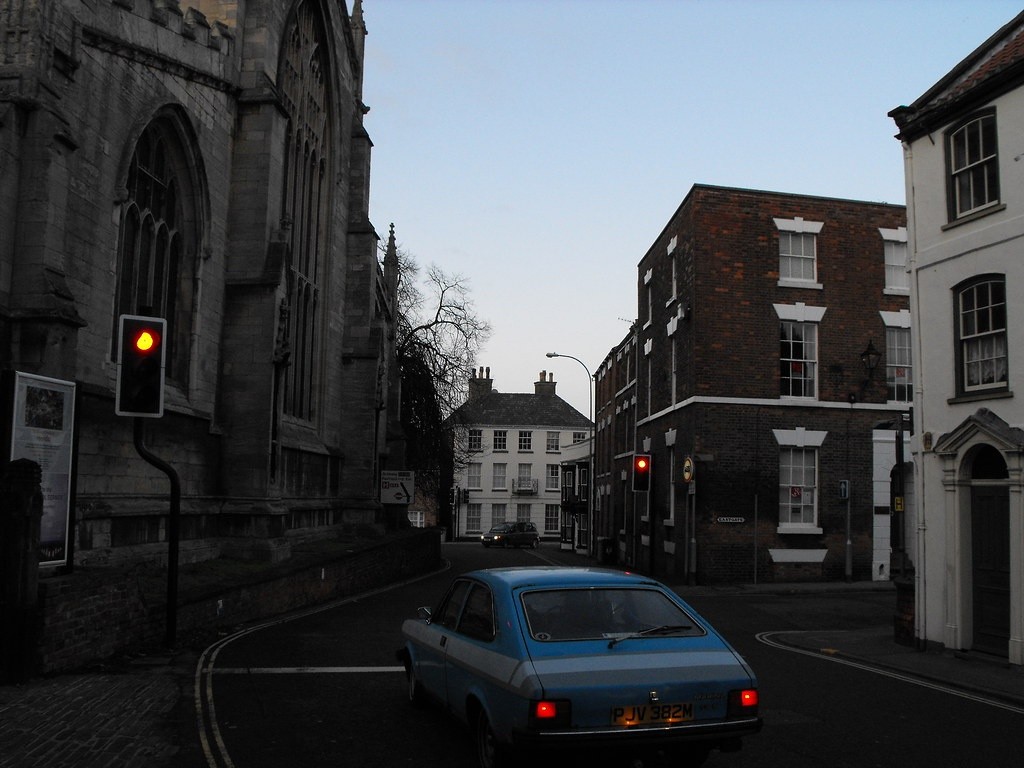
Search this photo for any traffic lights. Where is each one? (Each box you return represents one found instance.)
[116,314,167,418]
[632,452,651,495]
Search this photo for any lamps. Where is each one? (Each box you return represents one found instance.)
[860,337,882,401]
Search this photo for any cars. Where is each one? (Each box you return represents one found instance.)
[480,522,540,549]
[394,565,764,767]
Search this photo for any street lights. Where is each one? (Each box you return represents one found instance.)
[546,351,592,555]
